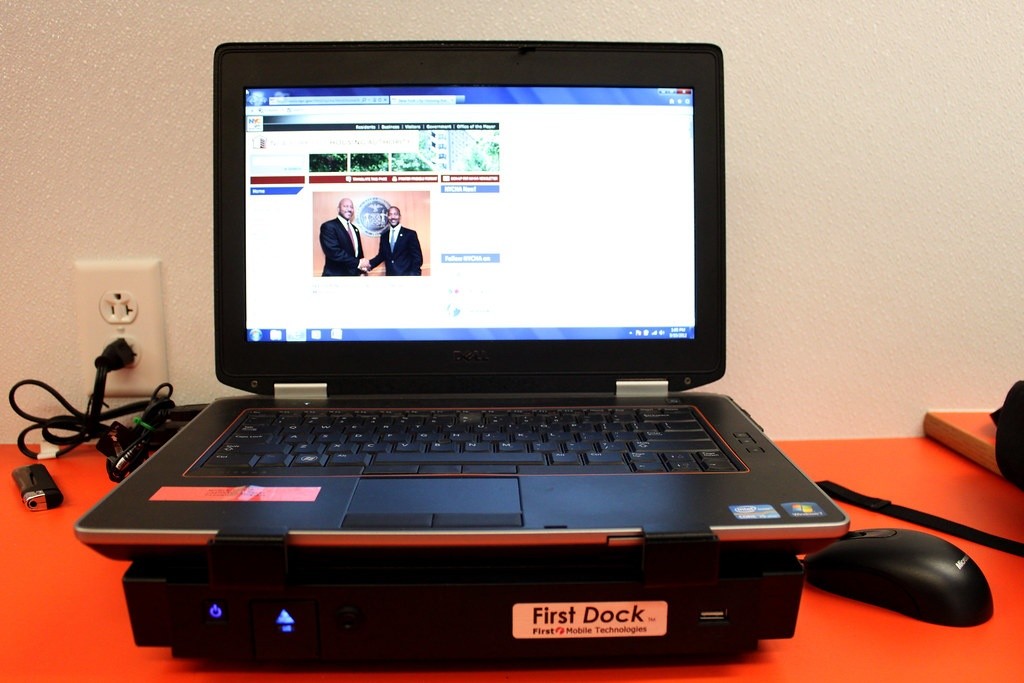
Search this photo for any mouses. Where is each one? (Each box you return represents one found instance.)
[801,527,993,629]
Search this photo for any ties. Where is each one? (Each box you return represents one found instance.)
[391,230,394,253]
[347,222,355,252]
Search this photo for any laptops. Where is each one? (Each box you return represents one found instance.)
[76,41,851,548]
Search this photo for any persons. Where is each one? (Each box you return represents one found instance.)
[362,206,423,276]
[320,198,371,276]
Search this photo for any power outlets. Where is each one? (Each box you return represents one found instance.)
[75,259,168,397]
